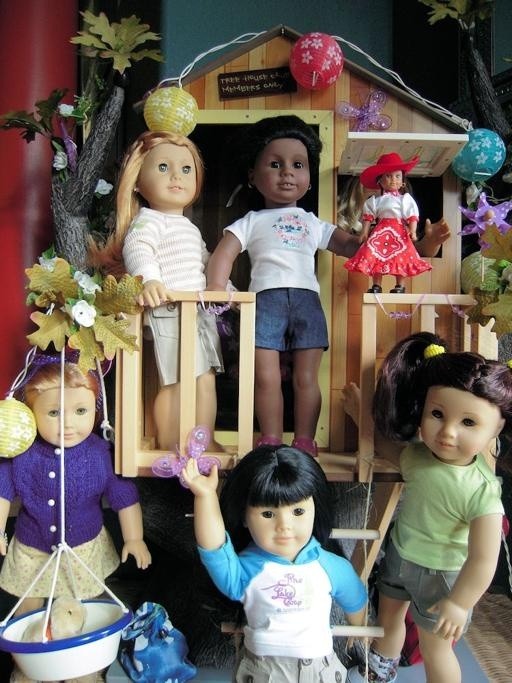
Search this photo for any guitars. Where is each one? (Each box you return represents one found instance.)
[452,128,506,182]
[143,86,198,137]
[0,400,37,458]
[289,31,344,91]
[462,250,511,296]
